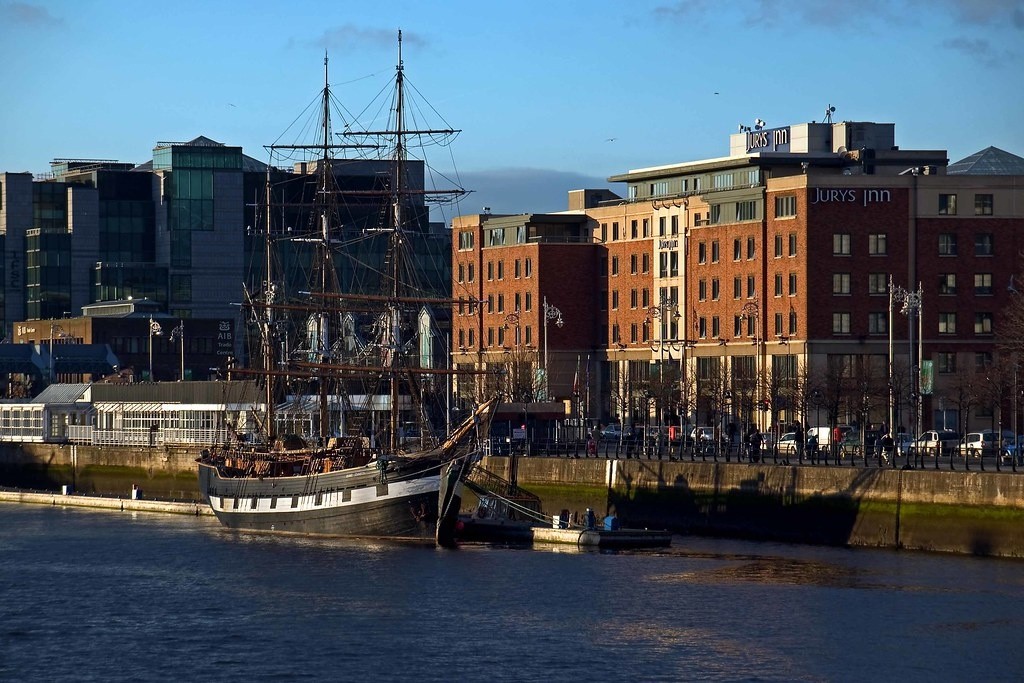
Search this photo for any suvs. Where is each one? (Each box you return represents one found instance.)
[913,428,960,459]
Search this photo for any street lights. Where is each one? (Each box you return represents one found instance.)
[907,281,922,444]
[169,319,184,382]
[888,274,911,444]
[543,295,565,403]
[49,320,66,384]
[503,304,521,386]
[659,295,682,456]
[150,321,162,382]
[740,300,760,435]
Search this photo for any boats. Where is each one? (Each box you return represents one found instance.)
[457,492,673,549]
[528,525,601,546]
[533,541,600,555]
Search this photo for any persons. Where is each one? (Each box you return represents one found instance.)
[727,419,737,441]
[625,428,644,459]
[587,425,600,441]
[696,427,704,457]
[865,420,872,430]
[874,435,883,467]
[880,420,889,432]
[881,434,893,465]
[897,422,906,433]
[850,420,857,426]
[969,444,978,462]
[807,434,818,465]
[788,420,800,433]
[749,429,764,463]
[793,425,804,458]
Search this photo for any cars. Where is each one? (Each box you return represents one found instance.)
[600,423,631,442]
[773,431,800,455]
[755,432,778,452]
[1002,434,1024,457]
[954,432,1008,458]
[879,432,915,456]
[690,427,731,445]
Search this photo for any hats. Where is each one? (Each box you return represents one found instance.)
[594,426,597,429]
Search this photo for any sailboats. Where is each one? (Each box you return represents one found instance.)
[193,25,506,543]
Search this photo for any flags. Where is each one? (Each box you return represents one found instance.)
[573,365,579,392]
[585,359,590,388]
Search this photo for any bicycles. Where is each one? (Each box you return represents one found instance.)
[873,442,895,470]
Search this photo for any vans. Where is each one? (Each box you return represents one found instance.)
[805,426,835,454]
